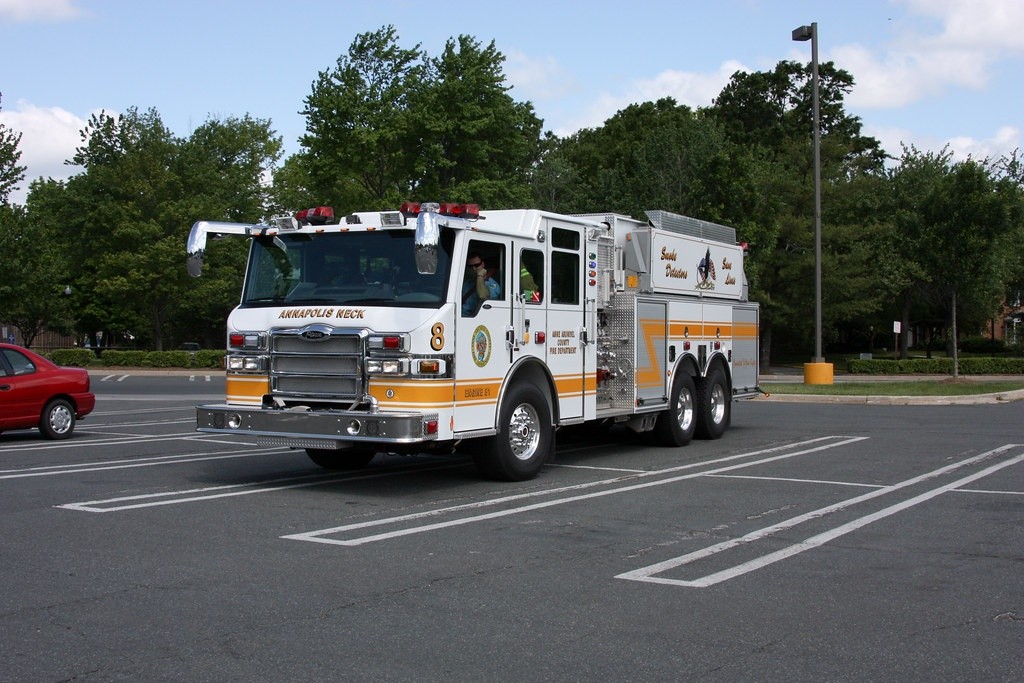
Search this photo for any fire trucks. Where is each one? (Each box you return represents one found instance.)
[186,202,761,482]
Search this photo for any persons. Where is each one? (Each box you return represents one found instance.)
[521,259,540,302]
[462,253,501,317]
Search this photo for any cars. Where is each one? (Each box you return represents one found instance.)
[0,341,96,441]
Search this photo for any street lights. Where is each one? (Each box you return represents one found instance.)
[791,21,828,362]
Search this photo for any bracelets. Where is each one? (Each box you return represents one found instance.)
[477,275,484,278]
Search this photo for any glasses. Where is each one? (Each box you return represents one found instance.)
[467,261,483,269]
